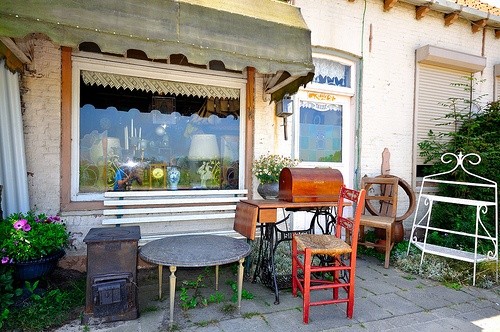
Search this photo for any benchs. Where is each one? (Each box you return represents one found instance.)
[101,189,266,247]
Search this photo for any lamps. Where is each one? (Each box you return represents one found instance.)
[276,94,294,141]
[188,134,220,189]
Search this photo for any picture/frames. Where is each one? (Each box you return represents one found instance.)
[151,96,177,125]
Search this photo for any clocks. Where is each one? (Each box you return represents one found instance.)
[149,163,167,190]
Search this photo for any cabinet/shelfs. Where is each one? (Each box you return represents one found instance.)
[406,151,498,288]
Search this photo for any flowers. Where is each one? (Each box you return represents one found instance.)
[0,206,73,264]
[250,152,302,184]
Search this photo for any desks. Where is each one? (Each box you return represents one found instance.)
[233,199,352,305]
[138,235,251,329]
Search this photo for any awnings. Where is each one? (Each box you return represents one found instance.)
[0,0,315,105]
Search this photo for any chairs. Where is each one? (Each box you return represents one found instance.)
[291,184,366,325]
[344,178,398,269]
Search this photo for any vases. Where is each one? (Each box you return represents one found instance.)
[257,181,280,200]
[375,221,404,244]
[167,165,181,190]
[15,250,65,284]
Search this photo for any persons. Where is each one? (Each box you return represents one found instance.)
[113,159,142,192]
